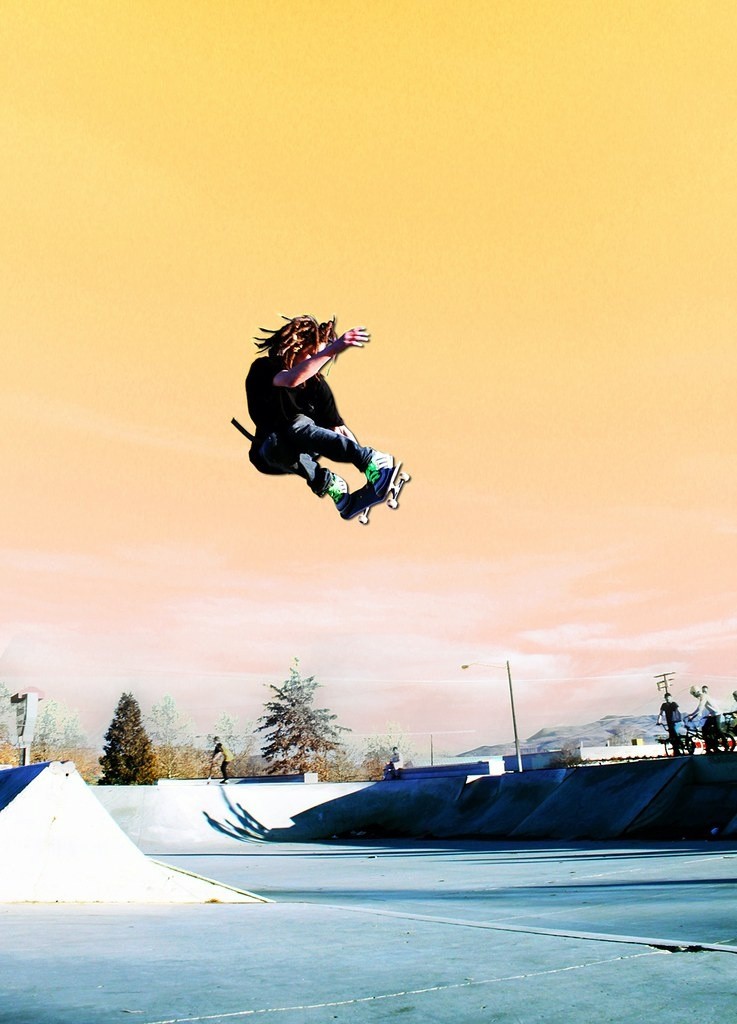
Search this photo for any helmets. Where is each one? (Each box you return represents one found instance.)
[690,685,701,696]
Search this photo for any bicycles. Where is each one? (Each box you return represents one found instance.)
[655,710,737,757]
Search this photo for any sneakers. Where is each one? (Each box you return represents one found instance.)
[325,472,353,518]
[363,451,395,498]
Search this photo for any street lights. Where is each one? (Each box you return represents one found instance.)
[460,659,523,773]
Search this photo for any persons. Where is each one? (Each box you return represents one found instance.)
[657,692,682,757]
[209,735,235,785]
[247,315,395,512]
[382,747,402,780]
[686,685,737,756]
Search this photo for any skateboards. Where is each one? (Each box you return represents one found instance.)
[341,461,412,525]
